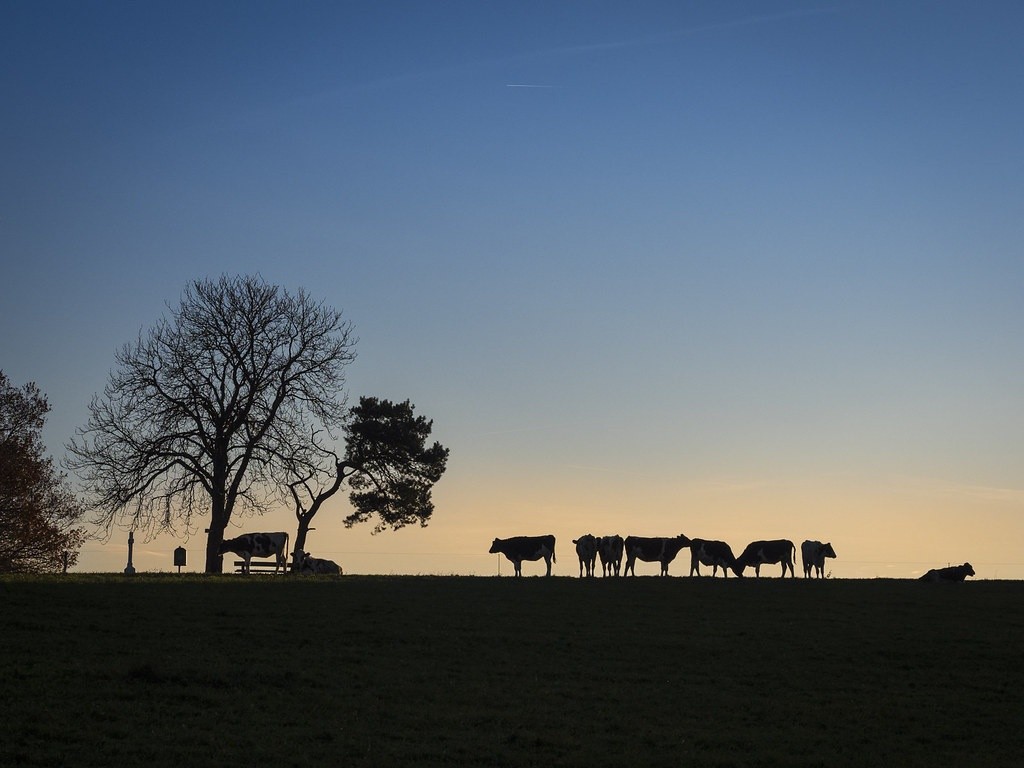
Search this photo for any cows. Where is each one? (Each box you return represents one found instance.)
[918,562,976,582]
[211,531,291,578]
[487,533,840,581]
[289,550,343,577]
